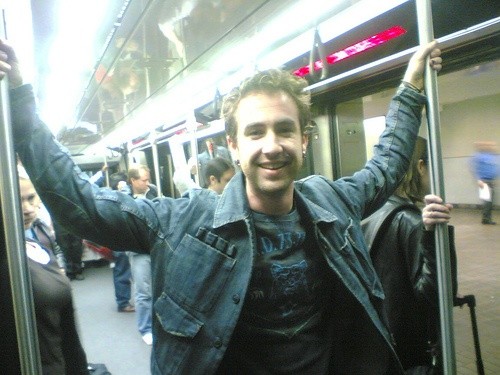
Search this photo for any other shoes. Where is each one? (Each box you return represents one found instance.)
[141,332,154,346]
[482,220,496,225]
[118,304,136,312]
[75,273,86,280]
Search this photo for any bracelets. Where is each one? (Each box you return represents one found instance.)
[400,79,422,93]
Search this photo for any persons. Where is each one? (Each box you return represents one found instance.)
[206,157,235,196]
[467,141,500,225]
[52,219,85,280]
[360,135,458,375]
[0,40,443,375]
[120,163,161,346]
[109,172,135,312]
[17,165,89,375]
